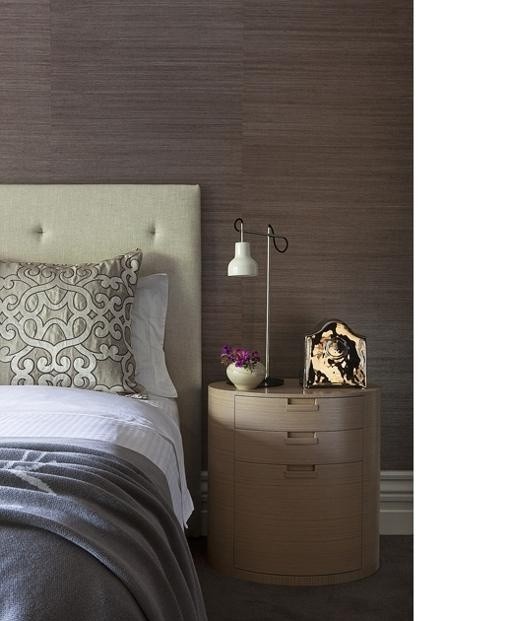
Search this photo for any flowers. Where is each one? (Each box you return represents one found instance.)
[219,342,261,372]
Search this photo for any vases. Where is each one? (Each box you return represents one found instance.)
[226,360,266,391]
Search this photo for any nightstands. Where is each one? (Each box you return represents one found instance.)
[205,381,380,587]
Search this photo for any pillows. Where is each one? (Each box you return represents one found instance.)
[1,247,182,402]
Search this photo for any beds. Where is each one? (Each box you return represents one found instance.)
[1,182,226,620]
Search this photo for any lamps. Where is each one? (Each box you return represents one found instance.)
[228,216,289,388]
